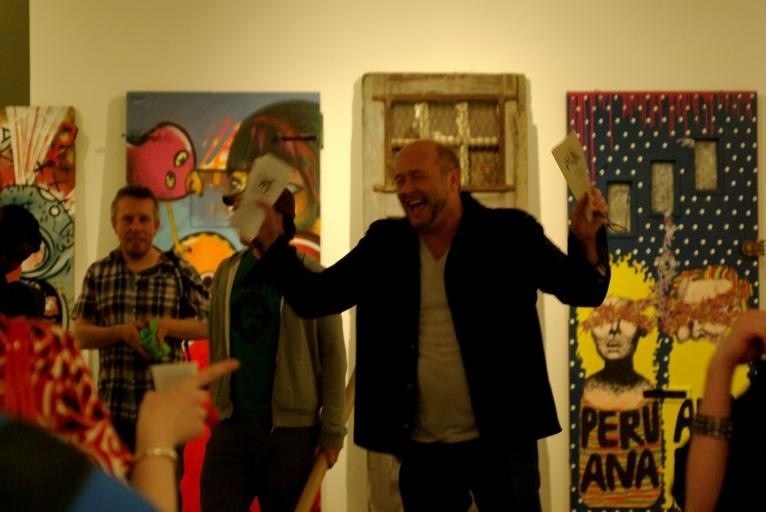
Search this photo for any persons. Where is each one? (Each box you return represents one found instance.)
[0,357,240,512]
[576,294,655,411]
[0,204,46,318]
[669,308,766,512]
[69,183,212,512]
[197,184,348,512]
[239,139,611,512]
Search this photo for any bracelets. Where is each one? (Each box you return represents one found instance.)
[134,447,179,461]
[692,413,734,441]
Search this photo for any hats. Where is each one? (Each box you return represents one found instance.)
[223,185,294,218]
